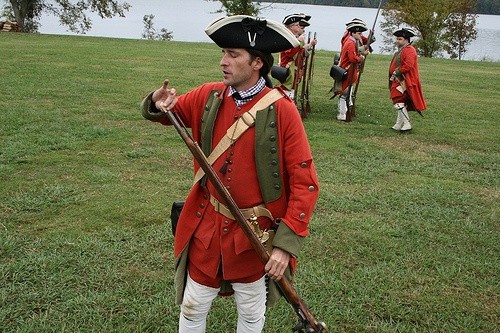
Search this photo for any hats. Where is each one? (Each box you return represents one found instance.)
[298,16,311,27]
[345,18,366,27]
[205,14,301,55]
[343,24,368,33]
[393,27,417,37]
[282,13,305,25]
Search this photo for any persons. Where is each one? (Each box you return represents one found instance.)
[141,15,320,333]
[388,28,428,132]
[279,12,376,122]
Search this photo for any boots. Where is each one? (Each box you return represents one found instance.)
[399,104,412,132]
[337,94,349,121]
[391,102,405,132]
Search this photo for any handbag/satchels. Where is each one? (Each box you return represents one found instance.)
[329,65,347,81]
[270,66,291,83]
[171,201,187,237]
[334,53,340,64]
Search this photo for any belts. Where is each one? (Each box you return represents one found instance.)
[209,196,274,222]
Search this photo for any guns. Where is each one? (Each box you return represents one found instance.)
[343,1,385,122]
[304,31,319,116]
[300,31,306,115]
[157,99,331,333]
